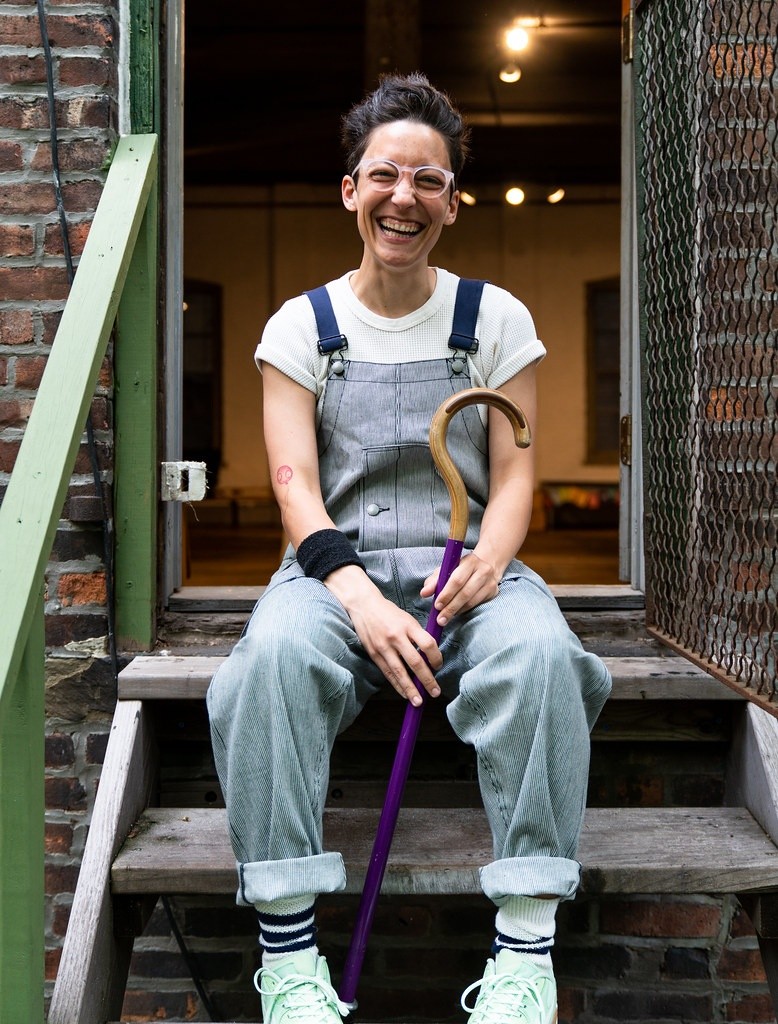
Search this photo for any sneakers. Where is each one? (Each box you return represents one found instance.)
[461,947,558,1024]
[253,950,350,1024]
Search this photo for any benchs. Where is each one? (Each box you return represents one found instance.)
[184,484,282,577]
[532,474,619,533]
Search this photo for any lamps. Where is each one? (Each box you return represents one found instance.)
[460,169,485,206]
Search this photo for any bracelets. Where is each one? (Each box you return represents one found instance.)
[295,527,366,582]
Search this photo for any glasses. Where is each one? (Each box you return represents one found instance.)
[350,159,456,199]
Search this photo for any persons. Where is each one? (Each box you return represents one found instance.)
[205,69,610,1024]
